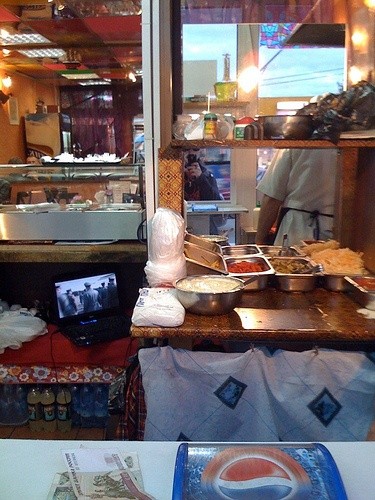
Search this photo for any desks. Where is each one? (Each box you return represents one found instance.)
[183,200,249,236]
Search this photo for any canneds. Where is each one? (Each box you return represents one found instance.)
[203,114,217,140]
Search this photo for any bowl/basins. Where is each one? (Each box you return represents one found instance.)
[171,233,375,315]
[254,114,316,139]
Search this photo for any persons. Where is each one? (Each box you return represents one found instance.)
[55,277,118,317]
[183,148,227,235]
[254,149,339,248]
[23,156,50,192]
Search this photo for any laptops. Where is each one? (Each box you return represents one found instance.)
[49,269,132,347]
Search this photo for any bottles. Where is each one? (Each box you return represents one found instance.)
[13,384,27,422]
[172,112,254,140]
[56,386,70,420]
[214,56,238,101]
[0,383,14,423]
[27,384,42,420]
[39,384,55,421]
[79,382,94,417]
[70,383,80,413]
[94,382,108,416]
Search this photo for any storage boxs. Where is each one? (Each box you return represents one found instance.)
[1,164,147,240]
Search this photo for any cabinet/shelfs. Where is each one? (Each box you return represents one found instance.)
[129,141,375,356]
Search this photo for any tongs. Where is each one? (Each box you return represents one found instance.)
[292,264,323,274]
[278,233,290,257]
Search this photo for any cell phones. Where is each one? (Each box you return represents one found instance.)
[187,153,196,166]
